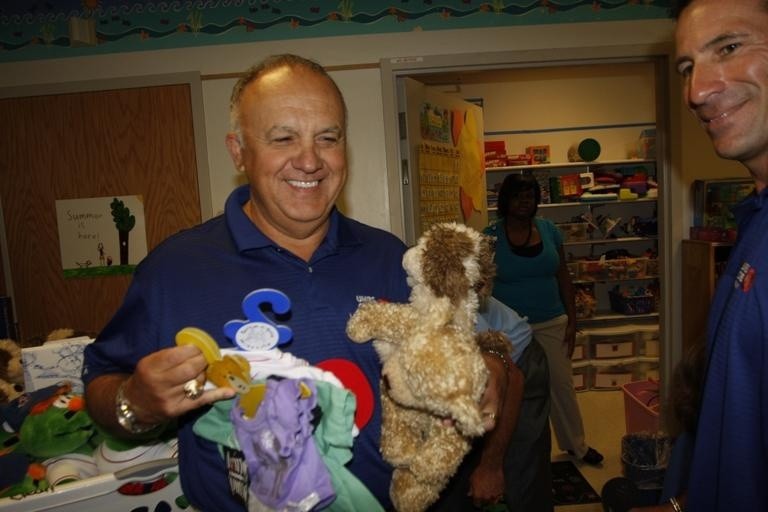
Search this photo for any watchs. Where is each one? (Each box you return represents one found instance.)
[114,375,158,434]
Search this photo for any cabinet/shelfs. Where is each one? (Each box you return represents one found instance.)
[485,159,660,391]
[680,237,736,424]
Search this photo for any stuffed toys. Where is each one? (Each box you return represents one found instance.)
[345,221,499,512]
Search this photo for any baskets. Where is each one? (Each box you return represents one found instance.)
[608,291,653,315]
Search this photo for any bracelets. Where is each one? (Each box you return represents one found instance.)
[667,496,683,512]
[476,329,513,378]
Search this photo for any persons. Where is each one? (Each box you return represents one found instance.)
[85,53,422,512]
[473,286,554,512]
[667,0,767,511]
[478,172,604,467]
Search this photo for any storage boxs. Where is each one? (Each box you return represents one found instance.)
[620,379,659,433]
[0,458,197,512]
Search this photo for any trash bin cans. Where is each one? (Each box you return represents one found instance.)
[621,447,670,480]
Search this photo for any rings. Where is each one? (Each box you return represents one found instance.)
[182,378,204,398]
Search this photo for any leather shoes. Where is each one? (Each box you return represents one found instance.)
[569,447,603,464]
